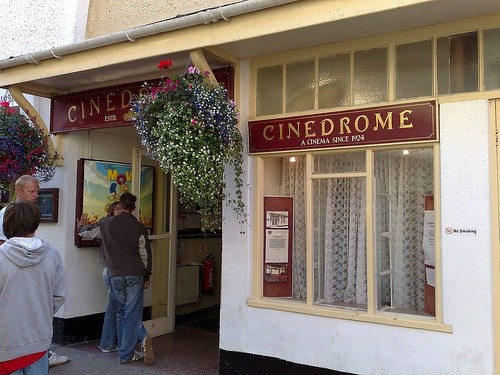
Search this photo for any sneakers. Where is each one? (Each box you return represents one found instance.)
[102,346,118,352]
[143,336,154,365]
[118,353,143,364]
[47,351,69,367]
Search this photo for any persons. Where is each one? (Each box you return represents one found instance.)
[99,200,130,354]
[78,191,155,365]
[0,202,67,375]
[0,172,69,368]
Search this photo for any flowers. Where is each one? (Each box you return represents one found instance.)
[0,93,60,196]
[130,61,253,238]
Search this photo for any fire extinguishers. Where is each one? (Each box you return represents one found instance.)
[202,256,213,294]
